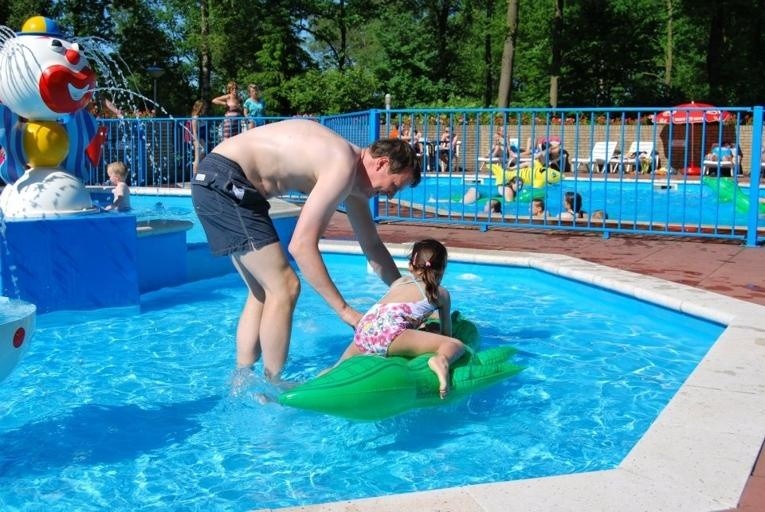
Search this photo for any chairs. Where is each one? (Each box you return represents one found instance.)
[414,138,430,171]
[703,143,741,176]
[435,141,462,173]
[478,138,659,174]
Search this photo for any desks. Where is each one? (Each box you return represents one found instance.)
[418,141,450,172]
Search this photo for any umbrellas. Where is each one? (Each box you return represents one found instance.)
[648,99,734,166]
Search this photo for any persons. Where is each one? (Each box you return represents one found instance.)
[187,118,422,384]
[391,122,423,154]
[241,84,268,128]
[497,177,524,202]
[317,238,467,400]
[531,198,550,218]
[211,81,246,141]
[708,140,732,162]
[439,125,458,172]
[485,198,502,214]
[102,162,132,212]
[557,190,608,219]
[187,99,207,176]
[489,126,559,165]
